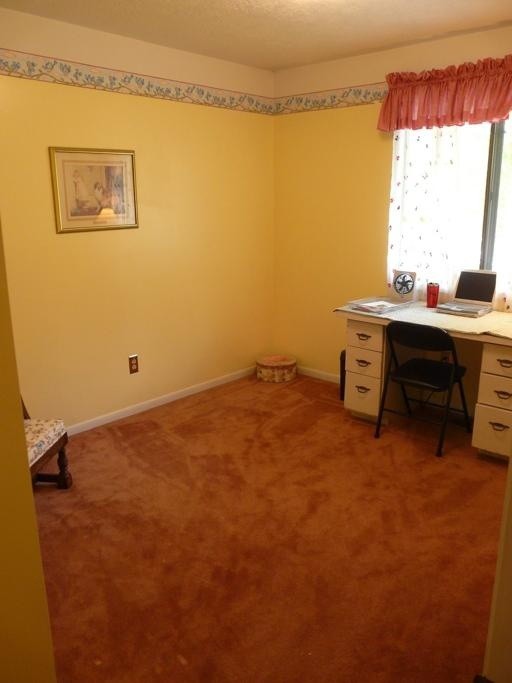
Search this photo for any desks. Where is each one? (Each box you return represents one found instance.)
[332,296,512,461]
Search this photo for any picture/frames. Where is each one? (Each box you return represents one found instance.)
[48,146,140,234]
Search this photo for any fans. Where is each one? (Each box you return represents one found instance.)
[391,269,417,302]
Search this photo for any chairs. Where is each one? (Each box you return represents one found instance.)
[19,395,73,489]
[374,321,473,457]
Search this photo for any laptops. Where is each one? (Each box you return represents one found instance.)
[436,269,499,318]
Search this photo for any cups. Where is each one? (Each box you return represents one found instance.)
[427,282,439,307]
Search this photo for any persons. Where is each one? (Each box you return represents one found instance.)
[68,169,88,209]
[95,181,108,207]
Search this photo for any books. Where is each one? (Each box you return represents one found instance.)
[349,297,398,314]
